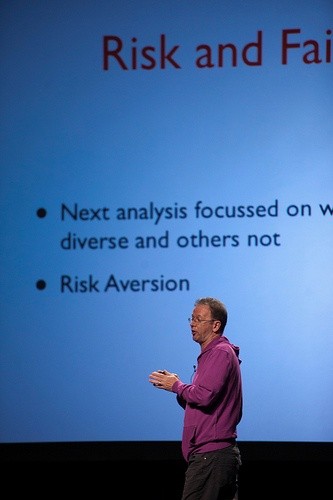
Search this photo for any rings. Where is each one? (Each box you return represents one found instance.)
[159,381,161,385]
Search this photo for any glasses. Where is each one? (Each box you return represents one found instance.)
[188,318,215,323]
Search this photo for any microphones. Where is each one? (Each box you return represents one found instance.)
[193,365,197,373]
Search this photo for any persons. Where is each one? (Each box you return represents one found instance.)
[148,296,243,500]
